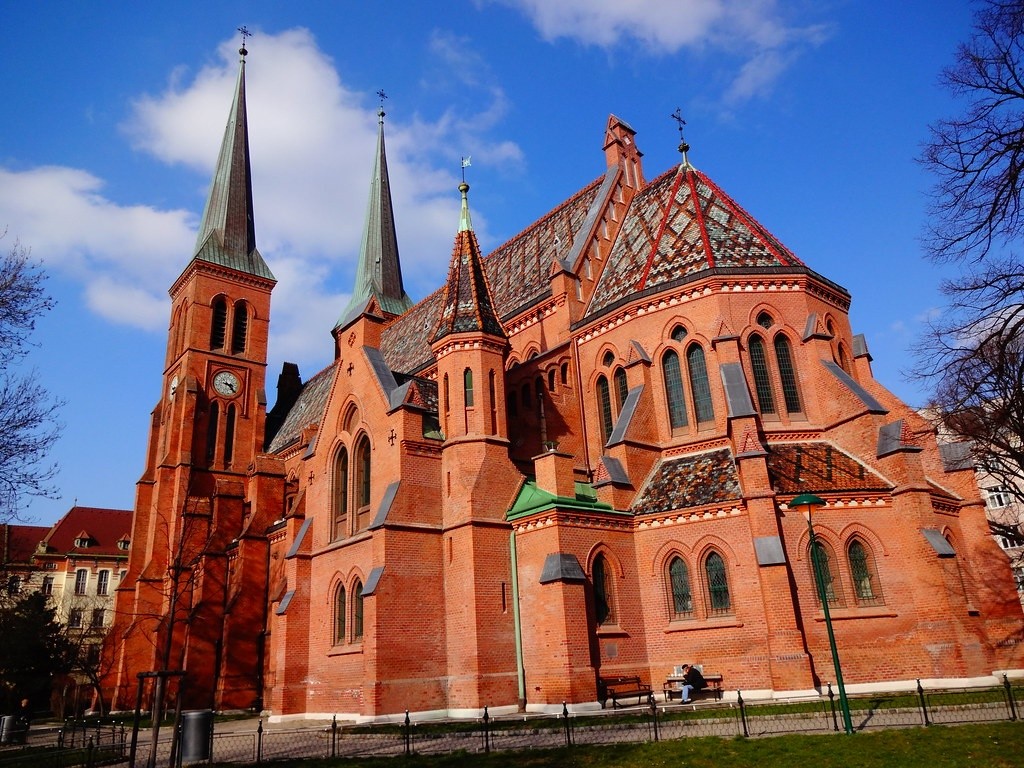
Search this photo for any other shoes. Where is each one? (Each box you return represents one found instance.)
[679,700,690,705]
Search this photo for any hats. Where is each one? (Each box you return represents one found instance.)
[682,664,688,669]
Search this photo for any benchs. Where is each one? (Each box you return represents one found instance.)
[663,675,724,702]
[598,675,654,710]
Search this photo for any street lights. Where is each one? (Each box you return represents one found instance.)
[786,495,855,733]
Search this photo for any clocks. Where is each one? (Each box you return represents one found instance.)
[209,367,243,399]
[168,373,178,401]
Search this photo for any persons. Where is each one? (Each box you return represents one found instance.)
[15,698,32,744]
[680,664,707,705]
